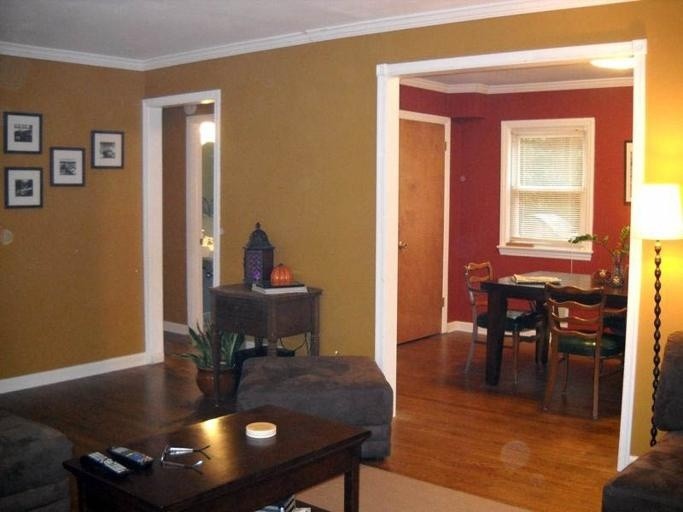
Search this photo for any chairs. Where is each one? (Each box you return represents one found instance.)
[541,277,624,420]
[462,259,547,388]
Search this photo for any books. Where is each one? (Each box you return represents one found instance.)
[510,275,563,284]
[251,280,308,295]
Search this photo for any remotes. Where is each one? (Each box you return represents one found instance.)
[107,446,154,466]
[87,451,131,476]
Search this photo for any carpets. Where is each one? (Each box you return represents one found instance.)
[286,460,534,512]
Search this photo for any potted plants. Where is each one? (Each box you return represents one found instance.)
[174,316,243,401]
[567,225,636,288]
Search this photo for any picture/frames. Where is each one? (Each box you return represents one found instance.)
[1,110,43,156]
[3,165,45,209]
[47,143,88,188]
[87,129,127,171]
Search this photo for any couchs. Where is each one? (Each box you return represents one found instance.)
[596,327,683,512]
[0,403,77,510]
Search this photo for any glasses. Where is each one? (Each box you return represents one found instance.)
[161,444,209,467]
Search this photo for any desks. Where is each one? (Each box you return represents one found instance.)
[206,279,325,411]
[61,402,373,512]
[476,266,625,389]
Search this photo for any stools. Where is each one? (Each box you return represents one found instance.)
[234,353,394,462]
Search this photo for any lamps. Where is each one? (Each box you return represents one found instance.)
[634,179,683,446]
[238,219,277,287]
